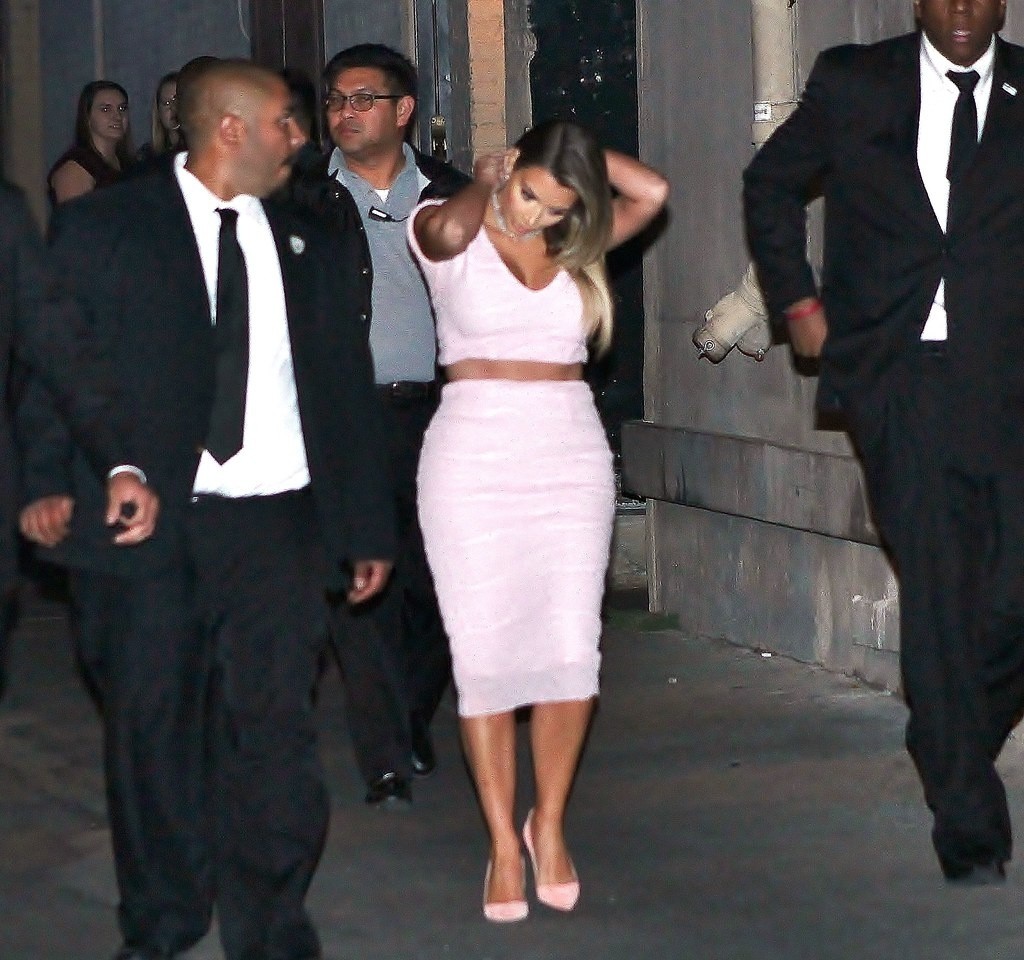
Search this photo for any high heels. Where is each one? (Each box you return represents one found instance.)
[482,854,528,922]
[521,807,581,910]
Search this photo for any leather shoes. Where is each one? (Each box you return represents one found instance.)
[362,773,413,815]
[408,725,436,773]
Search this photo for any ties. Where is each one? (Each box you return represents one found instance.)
[204,208,250,466]
[943,70,980,370]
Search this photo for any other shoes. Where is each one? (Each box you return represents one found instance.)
[933,828,1005,889]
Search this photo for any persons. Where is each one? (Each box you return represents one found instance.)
[0,43,641,960]
[403,122,672,922]
[740,0,1024,886]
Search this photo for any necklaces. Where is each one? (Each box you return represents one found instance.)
[491,192,543,240]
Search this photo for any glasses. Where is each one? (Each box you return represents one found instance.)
[324,93,405,111]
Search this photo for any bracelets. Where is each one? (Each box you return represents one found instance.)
[108,463,148,484]
[785,301,823,319]
